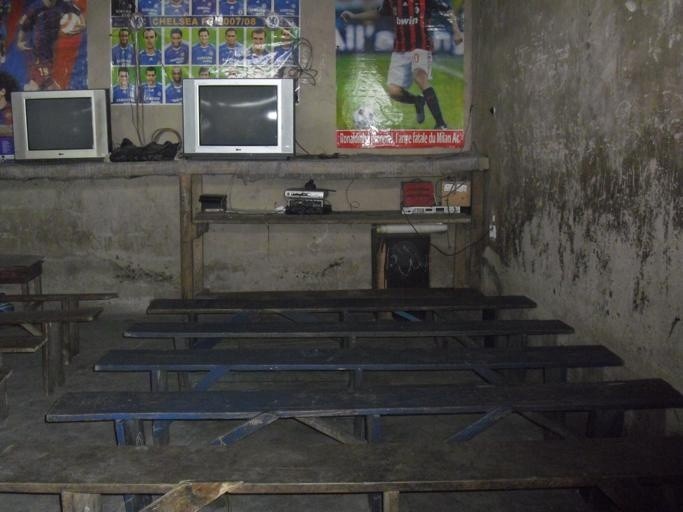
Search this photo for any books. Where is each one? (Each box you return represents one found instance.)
[441,176,471,207]
[199,194,227,214]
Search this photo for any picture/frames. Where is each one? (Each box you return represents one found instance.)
[0,252,44,309]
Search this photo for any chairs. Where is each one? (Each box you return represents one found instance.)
[434,124,448,130]
[416,95,426,123]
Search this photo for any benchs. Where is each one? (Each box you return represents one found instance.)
[0,284,683,395]
[2,437,682,509]
[47,378,683,449]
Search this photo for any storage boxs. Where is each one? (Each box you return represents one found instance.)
[441,176,471,207]
[199,194,227,214]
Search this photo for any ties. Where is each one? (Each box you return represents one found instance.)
[183,78,294,161]
[11,89,112,160]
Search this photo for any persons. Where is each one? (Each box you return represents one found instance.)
[111,0,297,105]
[338,0,464,131]
[0,71,23,125]
[16,0,84,91]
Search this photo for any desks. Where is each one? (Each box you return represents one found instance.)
[0,252,44,309]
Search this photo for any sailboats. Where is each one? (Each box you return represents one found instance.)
[353,106,376,128]
[60,12,81,35]
